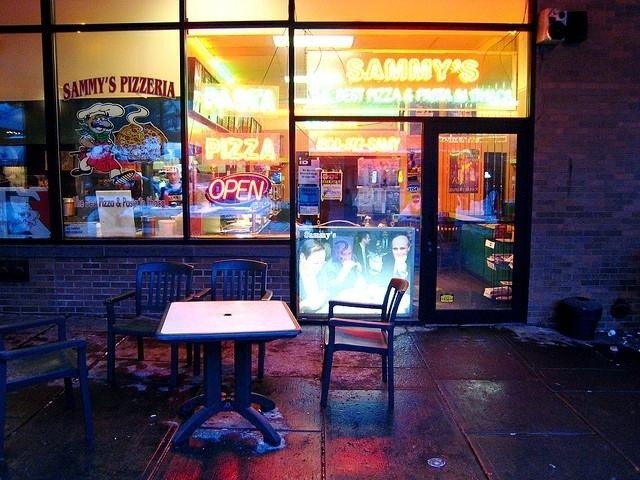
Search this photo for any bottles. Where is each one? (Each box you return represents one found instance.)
[140,189,170,206]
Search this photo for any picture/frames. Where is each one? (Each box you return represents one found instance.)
[296,226,417,320]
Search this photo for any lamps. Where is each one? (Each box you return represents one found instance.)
[270,30,357,54]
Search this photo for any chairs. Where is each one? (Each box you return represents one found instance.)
[192,257,271,383]
[439,219,464,275]
[1,304,96,457]
[316,278,410,416]
[103,260,197,392]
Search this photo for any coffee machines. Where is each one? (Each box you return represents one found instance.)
[73,150,143,218]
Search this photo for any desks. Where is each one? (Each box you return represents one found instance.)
[152,299,306,455]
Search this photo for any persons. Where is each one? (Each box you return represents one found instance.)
[299,231,411,315]
[159,167,182,206]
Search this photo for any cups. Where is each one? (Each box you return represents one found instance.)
[157,218,176,239]
[187,204,204,236]
[201,210,221,235]
[141,215,156,236]
